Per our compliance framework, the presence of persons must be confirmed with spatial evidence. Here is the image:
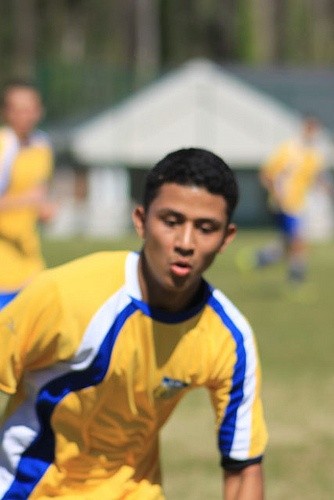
[257,114,334,280]
[0,148,268,500]
[0,75,59,311]
